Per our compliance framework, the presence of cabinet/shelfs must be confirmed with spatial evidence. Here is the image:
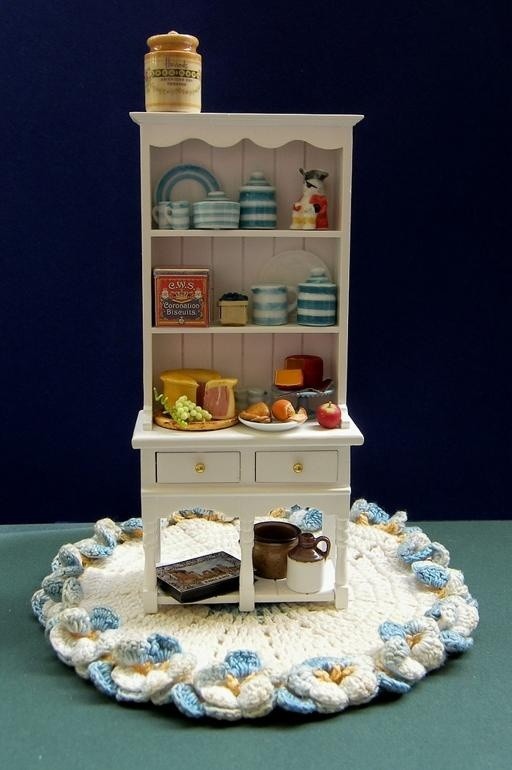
[125,106,369,620]
